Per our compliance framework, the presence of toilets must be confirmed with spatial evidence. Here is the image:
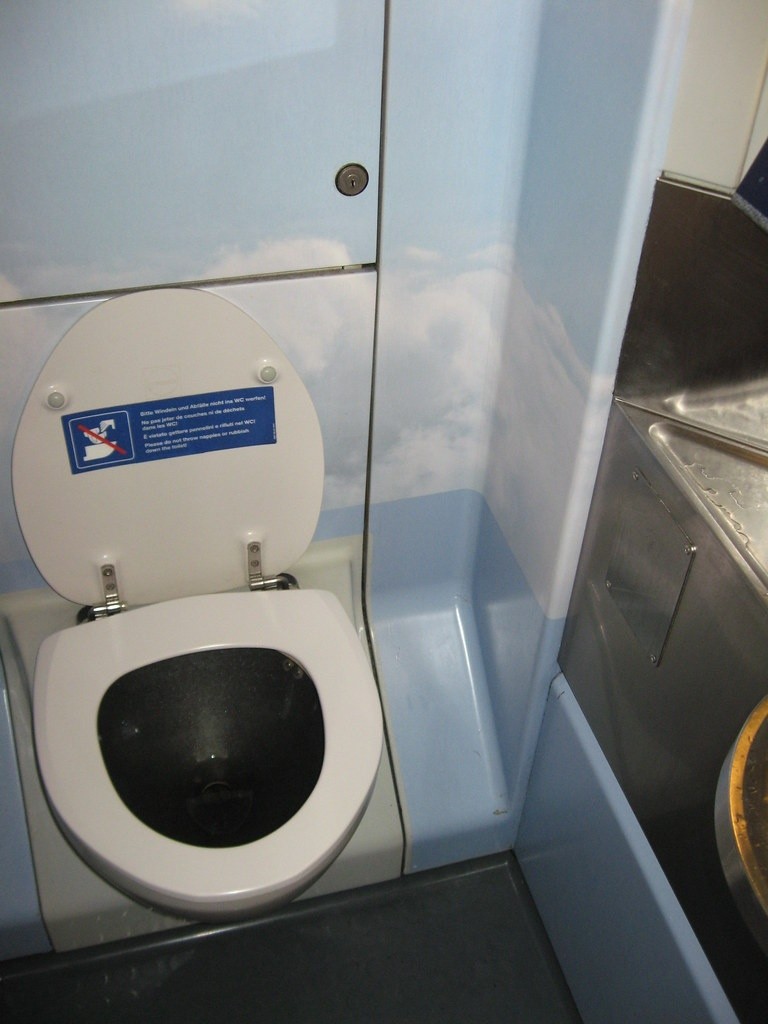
[0,282,385,919]
